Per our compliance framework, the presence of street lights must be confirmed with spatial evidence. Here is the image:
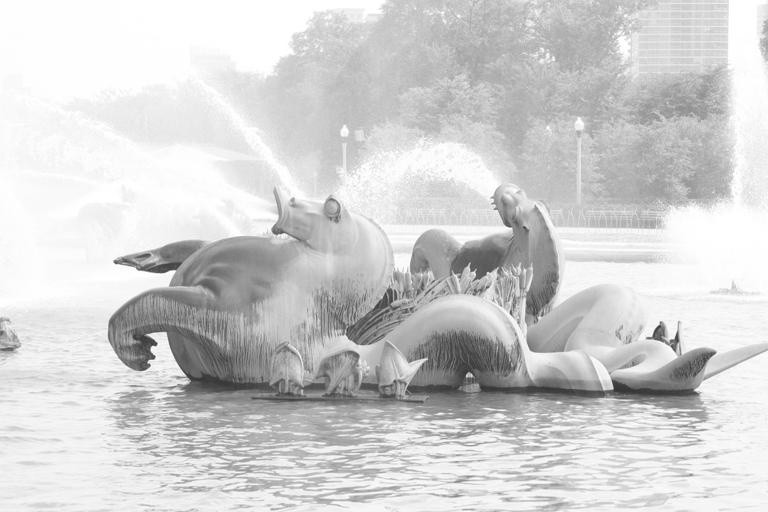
[339,124,349,179]
[544,125,554,200]
[574,117,585,208]
